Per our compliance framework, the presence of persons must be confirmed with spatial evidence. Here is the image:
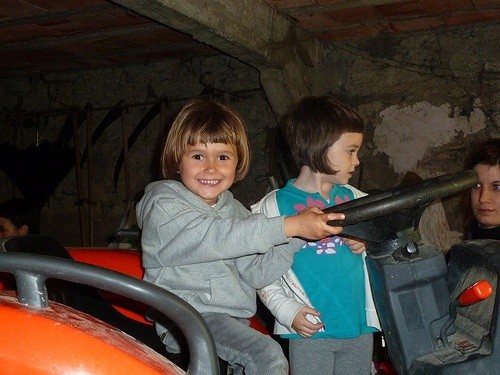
[0,202,119,319]
[134,99,345,375]
[250,94,387,375]
[462,139,500,240]
